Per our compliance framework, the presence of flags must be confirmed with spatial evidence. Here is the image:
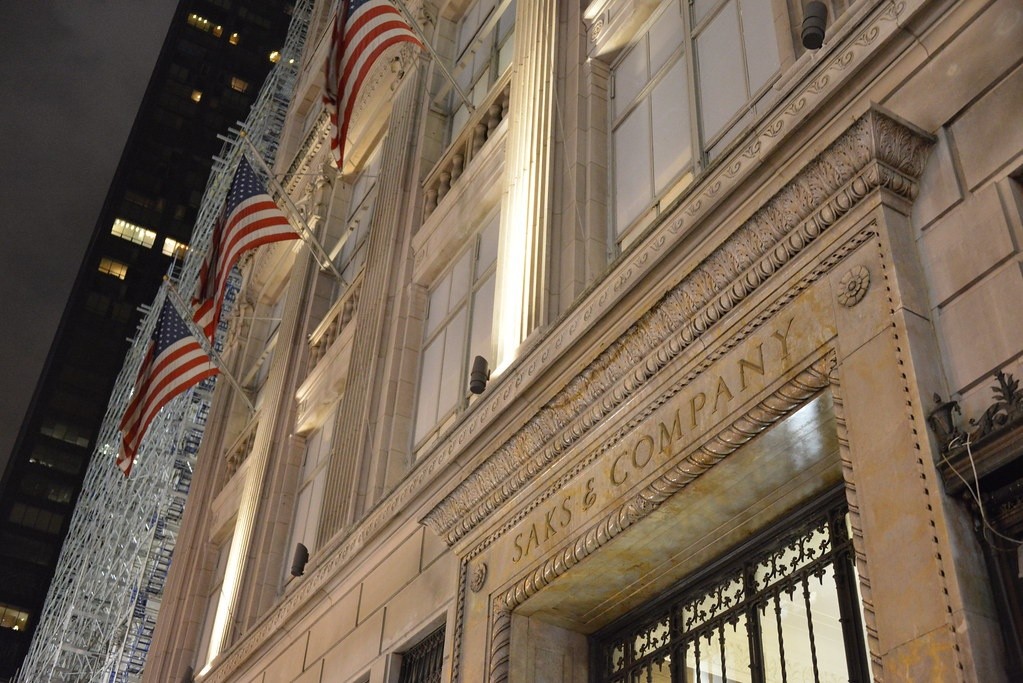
[321,0,427,172]
[116,293,219,478]
[190,153,300,347]
